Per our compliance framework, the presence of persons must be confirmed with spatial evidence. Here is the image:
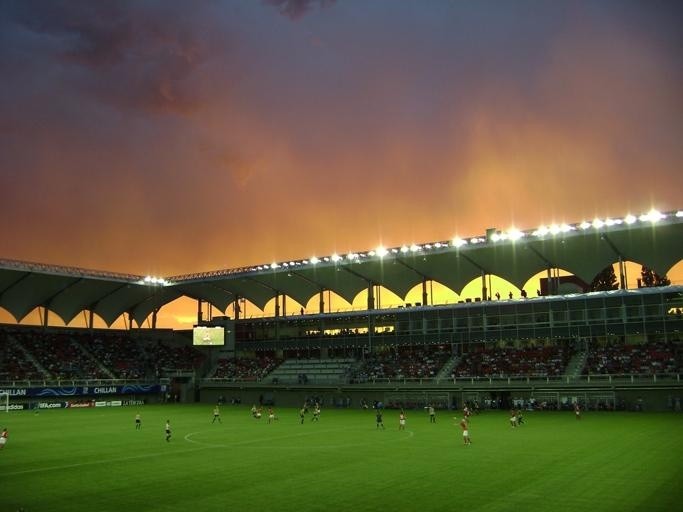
[0,323,200,407]
[508,408,516,427]
[309,405,320,422]
[398,410,407,431]
[209,405,222,424]
[164,419,171,442]
[516,409,524,426]
[267,406,278,424]
[374,409,384,430]
[134,412,141,430]
[447,336,683,418]
[209,331,453,411]
[451,417,471,446]
[0,427,8,450]
[298,405,306,424]
[427,406,436,423]
